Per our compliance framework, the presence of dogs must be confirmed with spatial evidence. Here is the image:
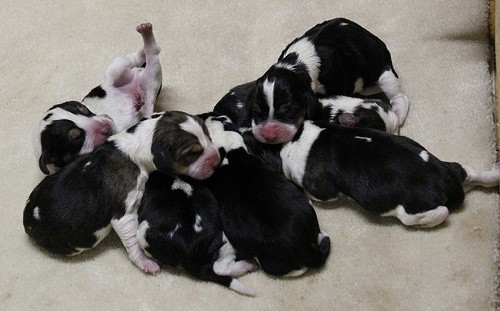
[22,18,500,297]
